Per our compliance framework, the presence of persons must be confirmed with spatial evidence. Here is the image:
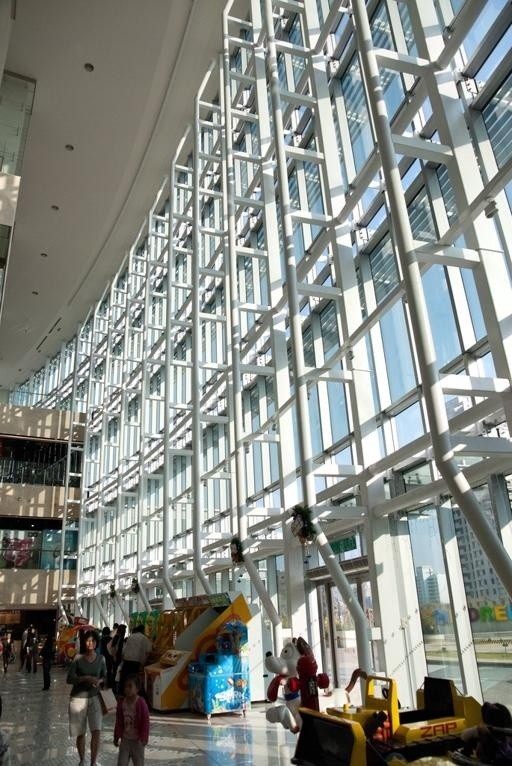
[98,622,152,695]
[0,693,11,765]
[1,621,42,675]
[38,628,57,691]
[66,629,107,765]
[461,700,512,766]
[113,670,149,766]
